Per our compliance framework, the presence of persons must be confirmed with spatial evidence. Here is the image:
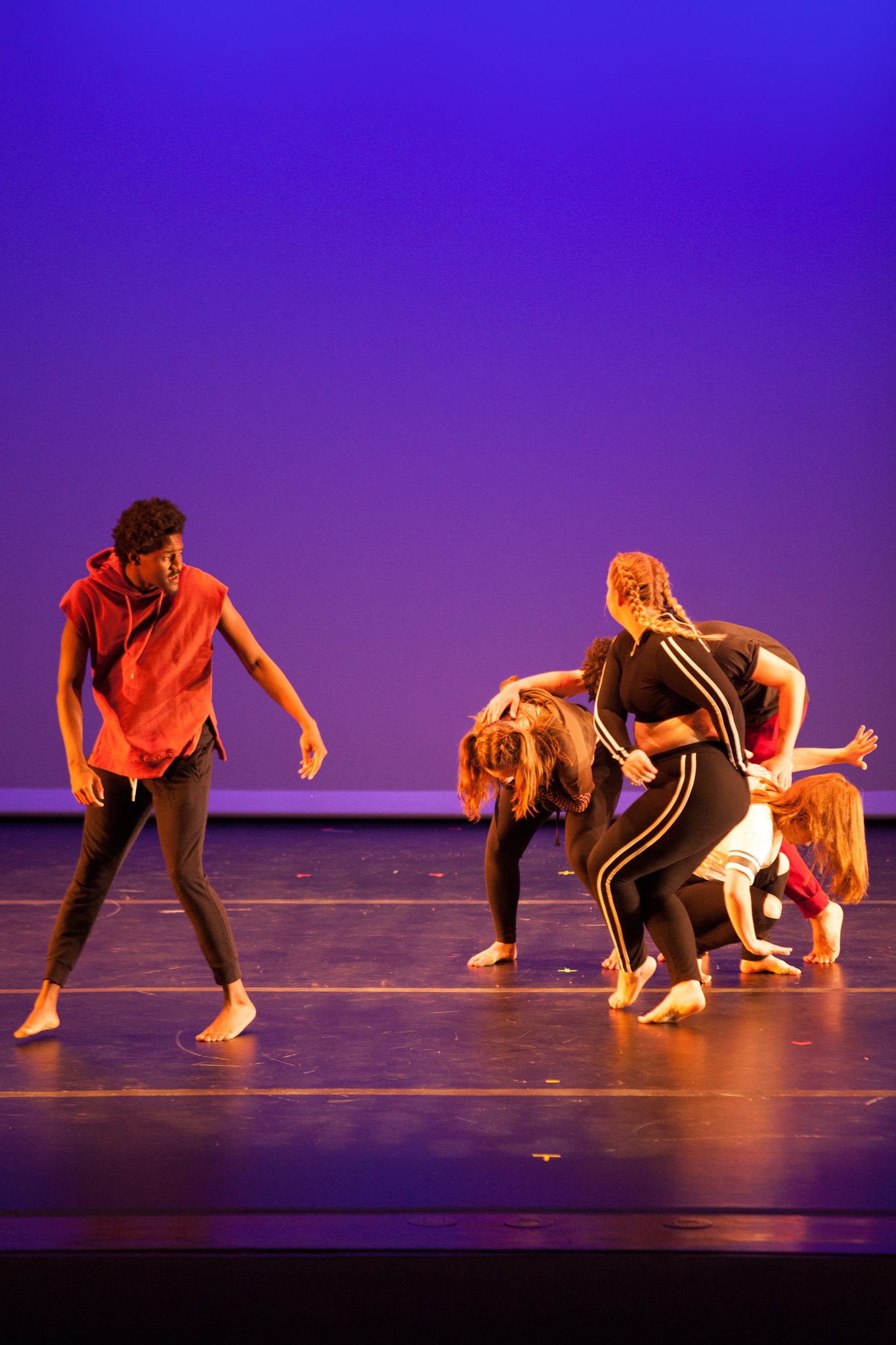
[456,675,623,970]
[13,497,327,1042]
[579,621,843,965]
[676,725,878,984]
[588,552,772,1025]
